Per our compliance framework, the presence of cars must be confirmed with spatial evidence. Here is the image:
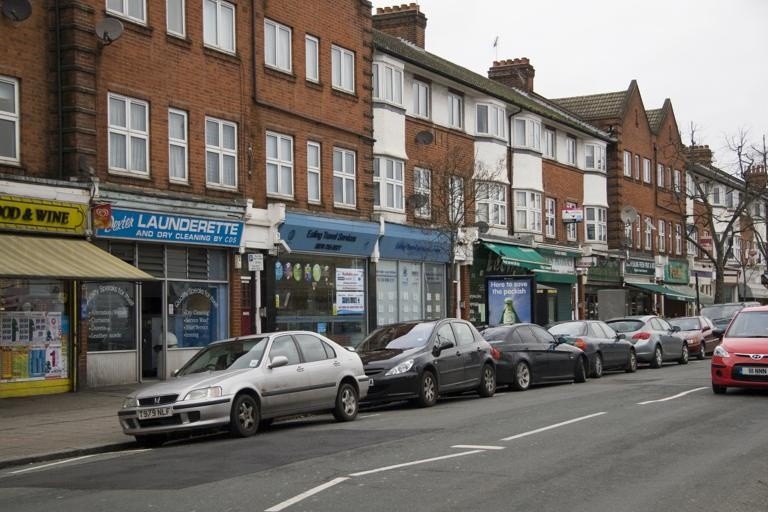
[547,321,636,377]
[667,316,722,357]
[352,319,499,405]
[480,321,587,389]
[606,315,688,371]
[119,330,372,435]
[705,302,768,394]
